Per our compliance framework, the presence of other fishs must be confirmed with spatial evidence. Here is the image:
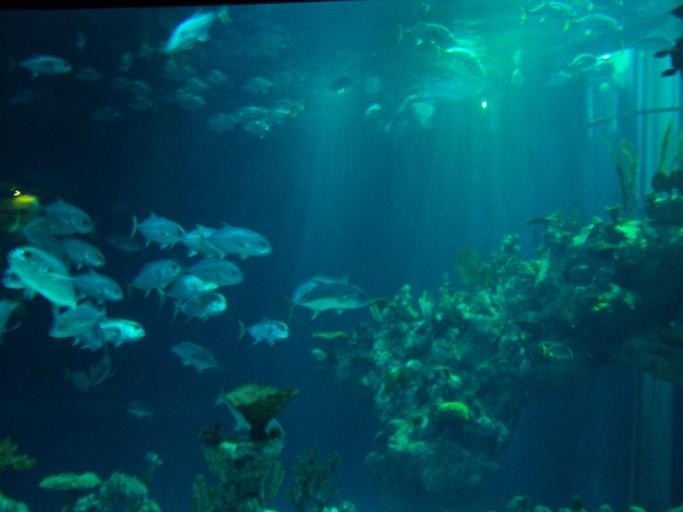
[0,185,274,350]
[1,0,682,140]
